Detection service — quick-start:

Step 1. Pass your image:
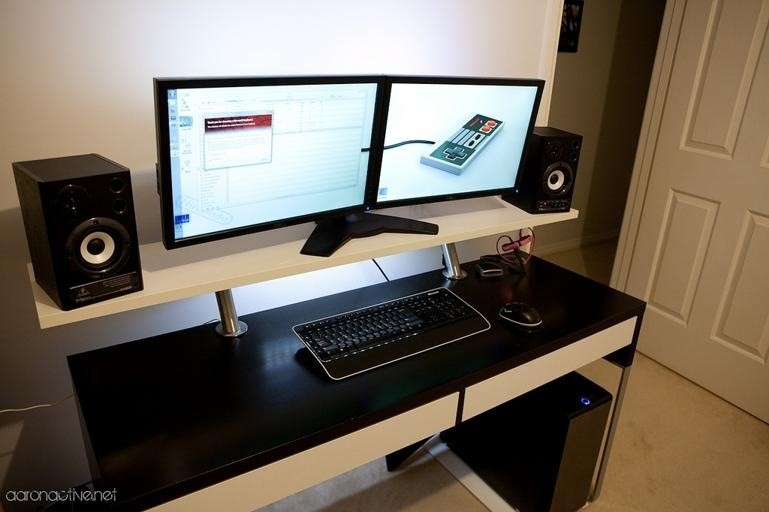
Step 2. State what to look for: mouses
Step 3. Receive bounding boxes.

[499,302,543,327]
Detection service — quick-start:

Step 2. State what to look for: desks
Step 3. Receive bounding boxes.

[26,194,647,512]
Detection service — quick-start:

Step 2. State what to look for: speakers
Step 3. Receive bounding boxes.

[12,153,143,311]
[502,127,583,214]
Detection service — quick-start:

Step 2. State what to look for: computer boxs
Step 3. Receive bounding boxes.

[440,370,613,512]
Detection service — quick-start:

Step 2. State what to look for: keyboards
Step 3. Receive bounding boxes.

[292,286,492,381]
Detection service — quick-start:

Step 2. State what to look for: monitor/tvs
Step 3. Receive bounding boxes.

[370,76,545,210]
[153,75,385,250]
